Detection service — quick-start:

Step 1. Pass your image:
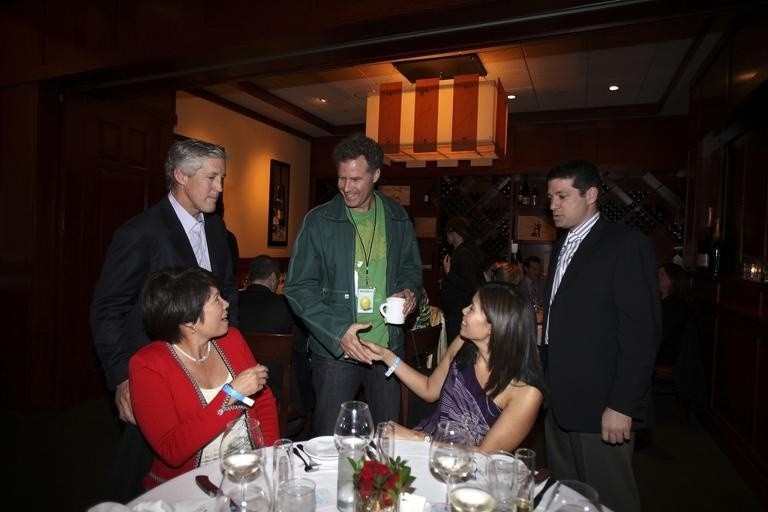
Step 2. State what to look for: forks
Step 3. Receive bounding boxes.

[296,443,320,467]
[292,448,319,473]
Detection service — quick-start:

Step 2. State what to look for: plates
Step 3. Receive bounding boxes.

[302,436,340,460]
[488,453,529,483]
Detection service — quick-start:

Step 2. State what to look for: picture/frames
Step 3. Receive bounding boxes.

[268,159,290,247]
[410,216,440,239]
[377,183,417,211]
[514,210,557,244]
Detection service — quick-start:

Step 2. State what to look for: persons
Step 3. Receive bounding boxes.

[442,219,546,347]
[344,281,544,454]
[657,262,699,374]
[89,140,238,502]
[128,268,282,492]
[542,160,662,512]
[282,133,423,435]
[239,255,316,419]
[412,288,429,331]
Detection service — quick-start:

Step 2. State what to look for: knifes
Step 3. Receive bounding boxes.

[195,476,238,512]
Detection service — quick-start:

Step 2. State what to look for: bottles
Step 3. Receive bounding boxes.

[272,183,285,229]
[692,205,723,277]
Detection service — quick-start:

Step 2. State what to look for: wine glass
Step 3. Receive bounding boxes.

[446,450,499,512]
[219,417,267,512]
[334,401,374,450]
[428,420,474,512]
[544,479,603,512]
[214,466,274,512]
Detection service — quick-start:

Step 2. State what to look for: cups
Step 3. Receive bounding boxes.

[376,423,395,465]
[485,451,518,512]
[278,477,316,512]
[336,448,363,512]
[515,447,536,512]
[272,439,294,512]
[379,296,406,325]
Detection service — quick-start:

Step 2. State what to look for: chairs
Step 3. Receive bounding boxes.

[402,324,441,429]
[649,323,709,434]
[237,333,295,435]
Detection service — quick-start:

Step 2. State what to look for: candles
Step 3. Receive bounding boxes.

[707,207,713,225]
[714,217,721,240]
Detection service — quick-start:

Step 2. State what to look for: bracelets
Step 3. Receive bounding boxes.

[385,356,401,377]
[222,384,244,402]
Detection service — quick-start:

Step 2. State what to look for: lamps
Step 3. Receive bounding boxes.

[366,53,509,169]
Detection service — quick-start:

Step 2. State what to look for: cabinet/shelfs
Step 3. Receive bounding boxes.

[688,275,768,512]
[435,172,515,313]
[595,172,690,267]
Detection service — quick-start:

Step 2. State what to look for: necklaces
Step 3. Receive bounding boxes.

[173,341,211,362]
